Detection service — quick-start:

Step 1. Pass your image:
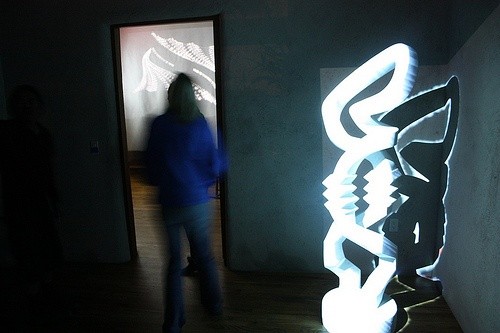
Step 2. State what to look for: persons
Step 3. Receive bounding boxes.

[128,66,229,331]
